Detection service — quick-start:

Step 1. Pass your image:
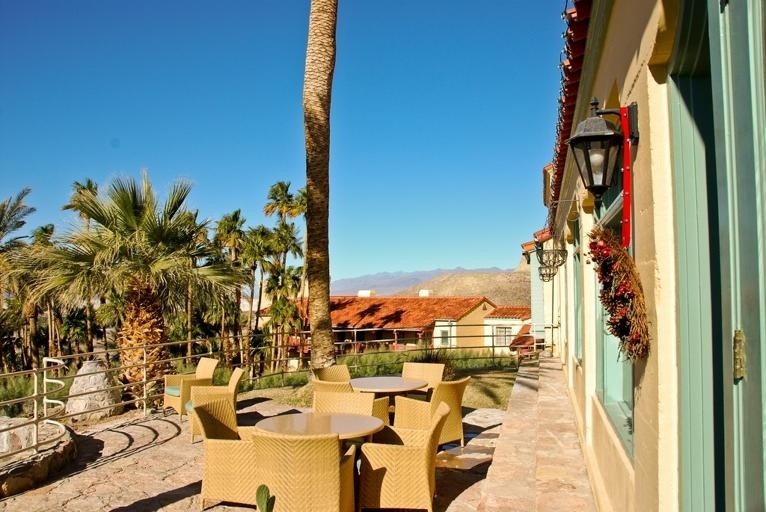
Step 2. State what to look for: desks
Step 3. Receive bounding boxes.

[254,413,383,450]
[348,377,429,407]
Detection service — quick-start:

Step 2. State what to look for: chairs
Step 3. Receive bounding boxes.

[399,361,445,399]
[312,378,391,425]
[190,399,256,512]
[185,367,245,443]
[359,402,452,512]
[249,432,356,512]
[311,364,349,381]
[393,375,471,448]
[161,356,221,423]
[310,391,374,444]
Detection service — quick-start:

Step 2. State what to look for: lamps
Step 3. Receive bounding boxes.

[567,97,639,207]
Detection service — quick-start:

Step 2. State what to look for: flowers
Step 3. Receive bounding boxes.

[584,218,650,363]
